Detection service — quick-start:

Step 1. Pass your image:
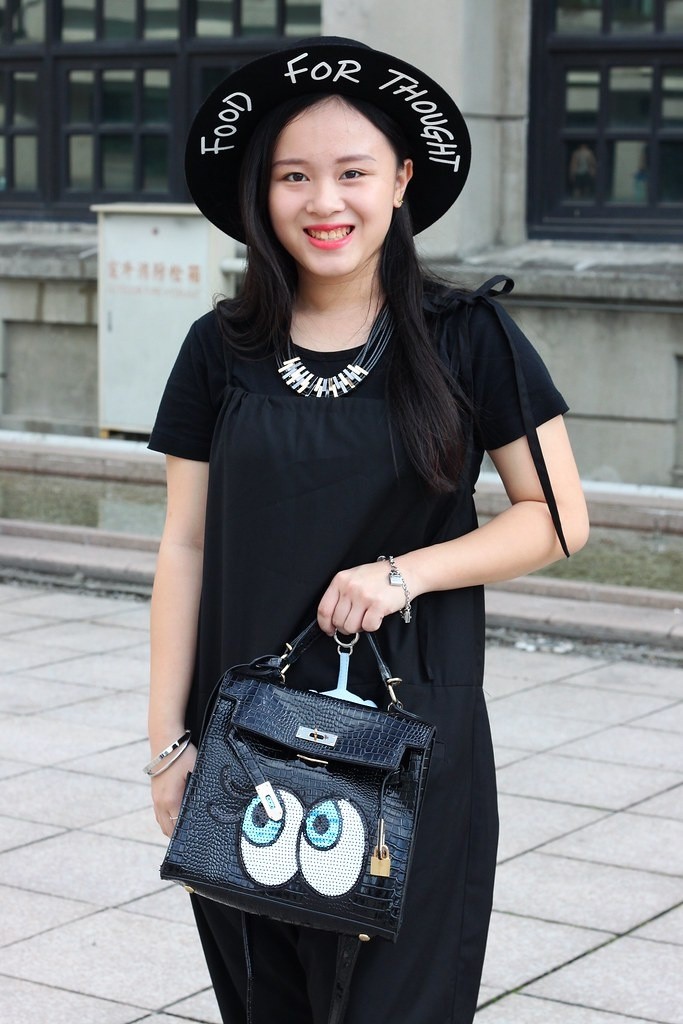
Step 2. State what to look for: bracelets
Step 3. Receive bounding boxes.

[378,553,411,624]
[143,729,192,778]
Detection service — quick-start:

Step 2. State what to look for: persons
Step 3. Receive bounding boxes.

[136,34,593,1024]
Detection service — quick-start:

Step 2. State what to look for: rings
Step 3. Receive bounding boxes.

[170,816,177,820]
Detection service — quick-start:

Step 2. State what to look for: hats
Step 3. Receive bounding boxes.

[184,36,472,247]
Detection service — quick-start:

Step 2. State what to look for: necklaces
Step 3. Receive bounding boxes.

[266,304,397,402]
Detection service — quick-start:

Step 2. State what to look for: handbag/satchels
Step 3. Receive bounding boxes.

[160,615,436,945]
